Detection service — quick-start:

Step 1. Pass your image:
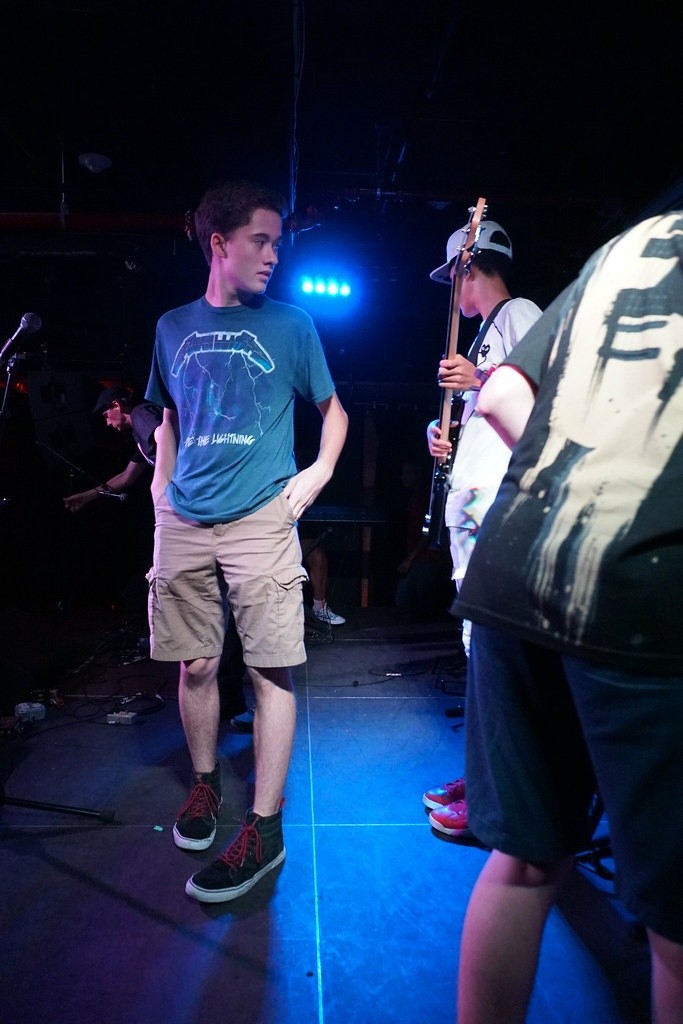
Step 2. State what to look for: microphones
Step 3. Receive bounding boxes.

[99,491,128,504]
[0,312,42,371]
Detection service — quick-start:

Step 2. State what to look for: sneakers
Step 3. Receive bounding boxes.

[172,758,223,851]
[429,799,472,836]
[422,778,465,810]
[312,602,346,625]
[184,808,286,903]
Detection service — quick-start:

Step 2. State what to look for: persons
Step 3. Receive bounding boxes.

[61,384,255,730]
[143,183,351,903]
[395,479,442,621]
[423,218,544,839]
[294,437,346,626]
[450,209,683,1024]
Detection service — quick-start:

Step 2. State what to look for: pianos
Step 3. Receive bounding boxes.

[297,502,394,528]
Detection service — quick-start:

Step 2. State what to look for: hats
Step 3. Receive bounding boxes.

[430,221,512,285]
[91,387,130,413]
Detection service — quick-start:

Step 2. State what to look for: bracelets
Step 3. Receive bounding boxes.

[94,488,100,496]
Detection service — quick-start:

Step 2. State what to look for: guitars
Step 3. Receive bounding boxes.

[419,197,489,553]
